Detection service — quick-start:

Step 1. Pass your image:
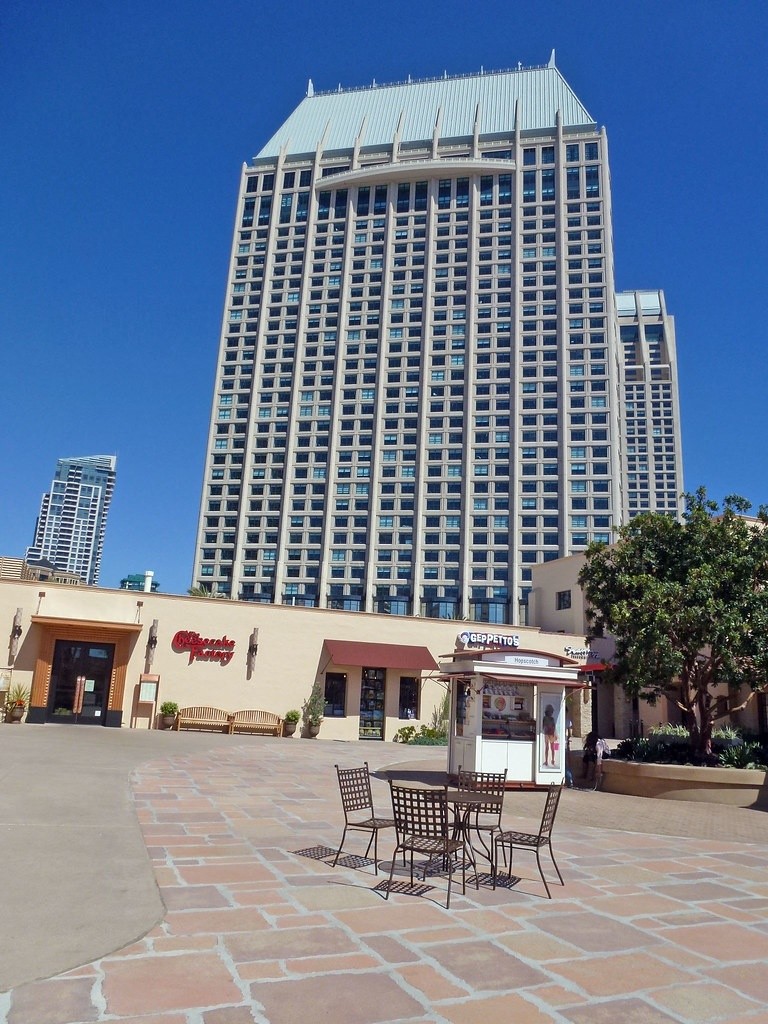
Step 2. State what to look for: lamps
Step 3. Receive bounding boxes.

[137,601,144,622]
[36,592,45,614]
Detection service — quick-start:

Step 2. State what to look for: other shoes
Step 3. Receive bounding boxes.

[543,762,548,766]
[551,761,554,765]
[576,785,593,791]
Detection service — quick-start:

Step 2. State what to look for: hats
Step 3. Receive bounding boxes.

[545,704,554,711]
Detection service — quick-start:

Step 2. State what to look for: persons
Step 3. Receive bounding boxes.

[583,732,611,790]
[565,705,574,788]
[543,704,558,766]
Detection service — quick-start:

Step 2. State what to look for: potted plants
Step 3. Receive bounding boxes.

[302,685,328,736]
[160,701,178,730]
[5,683,30,721]
[284,710,300,735]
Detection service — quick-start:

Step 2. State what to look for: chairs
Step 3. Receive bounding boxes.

[445,764,508,869]
[491,783,566,899]
[385,778,466,909]
[332,762,407,876]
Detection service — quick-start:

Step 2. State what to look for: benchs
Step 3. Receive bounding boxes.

[230,709,283,737]
[175,705,232,735]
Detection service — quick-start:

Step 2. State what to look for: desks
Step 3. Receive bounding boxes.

[423,791,503,890]
